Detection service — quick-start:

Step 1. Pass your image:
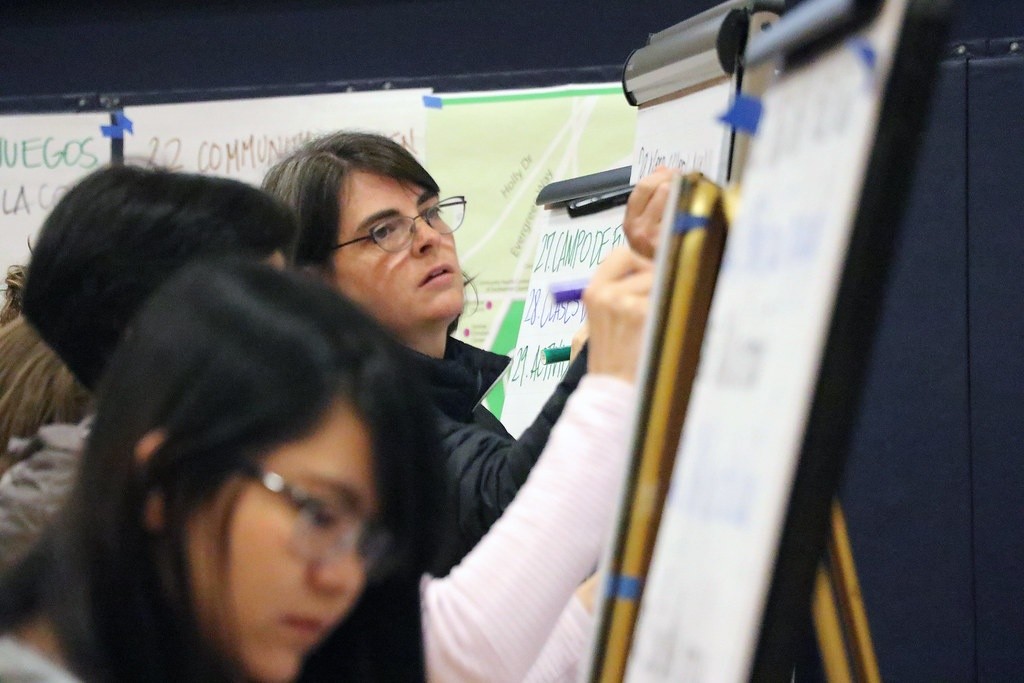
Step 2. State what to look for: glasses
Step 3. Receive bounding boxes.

[326,196,467,255]
[234,456,393,574]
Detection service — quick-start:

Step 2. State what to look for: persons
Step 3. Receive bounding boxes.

[260,132,680,576]
[0,264,437,683]
[19,162,657,683]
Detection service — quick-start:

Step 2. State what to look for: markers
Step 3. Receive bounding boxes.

[541,346,571,365]
[565,185,634,218]
[553,289,583,303]
